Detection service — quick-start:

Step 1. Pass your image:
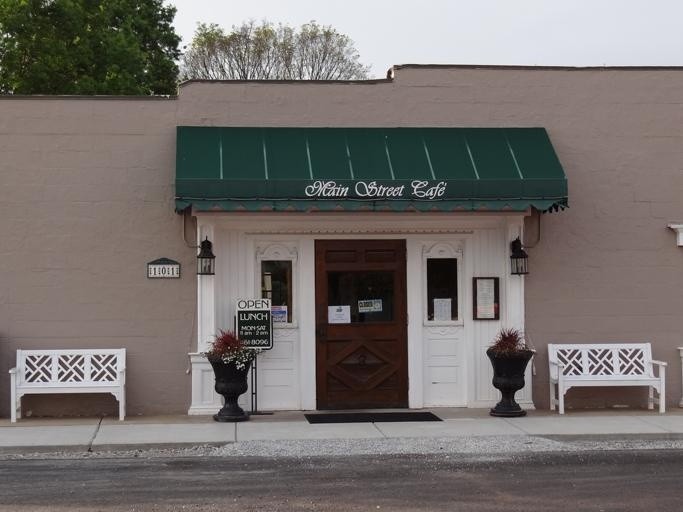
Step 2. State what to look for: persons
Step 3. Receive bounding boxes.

[337,307,342,312]
[359,302,370,307]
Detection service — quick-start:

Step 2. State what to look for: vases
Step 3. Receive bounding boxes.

[204,351,252,422]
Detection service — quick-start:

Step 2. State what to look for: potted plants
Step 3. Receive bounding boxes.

[485,326,534,416]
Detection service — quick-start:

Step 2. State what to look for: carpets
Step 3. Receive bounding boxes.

[302,411,442,423]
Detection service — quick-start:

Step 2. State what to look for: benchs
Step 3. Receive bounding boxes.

[547,342,668,413]
[7,348,131,426]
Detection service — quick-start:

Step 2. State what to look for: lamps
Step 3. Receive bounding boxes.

[196,234,216,276]
[510,236,529,275]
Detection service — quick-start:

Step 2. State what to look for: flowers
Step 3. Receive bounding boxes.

[200,328,257,372]
